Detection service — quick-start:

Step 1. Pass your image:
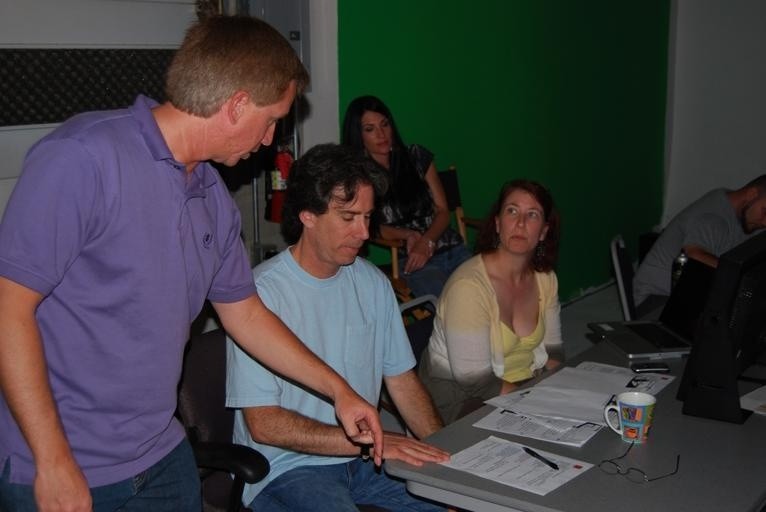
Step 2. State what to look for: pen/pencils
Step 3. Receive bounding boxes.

[522,446,559,470]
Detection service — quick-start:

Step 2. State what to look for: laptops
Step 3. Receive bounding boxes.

[588,258,716,360]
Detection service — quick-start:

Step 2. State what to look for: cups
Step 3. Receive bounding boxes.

[604,393,657,445]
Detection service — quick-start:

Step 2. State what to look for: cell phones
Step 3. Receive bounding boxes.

[631,362,669,373]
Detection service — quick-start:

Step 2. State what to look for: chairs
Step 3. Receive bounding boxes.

[400,293,439,372]
[365,164,470,319]
[610,233,636,322]
[175,324,270,512]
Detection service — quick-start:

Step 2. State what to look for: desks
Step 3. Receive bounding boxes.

[381,328,766,511]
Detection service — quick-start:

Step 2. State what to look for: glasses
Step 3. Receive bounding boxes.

[598,440,680,483]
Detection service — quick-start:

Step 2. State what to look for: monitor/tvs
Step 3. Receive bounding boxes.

[675,228,766,424]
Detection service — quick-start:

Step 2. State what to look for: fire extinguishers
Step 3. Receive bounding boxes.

[269,147,294,223]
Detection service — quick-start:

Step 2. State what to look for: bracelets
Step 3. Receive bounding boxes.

[360,439,370,462]
[420,235,439,250]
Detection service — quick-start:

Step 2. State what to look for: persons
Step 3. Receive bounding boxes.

[222,140,462,511]
[631,174,766,326]
[404,178,565,444]
[342,94,474,317]
[0,13,384,511]
